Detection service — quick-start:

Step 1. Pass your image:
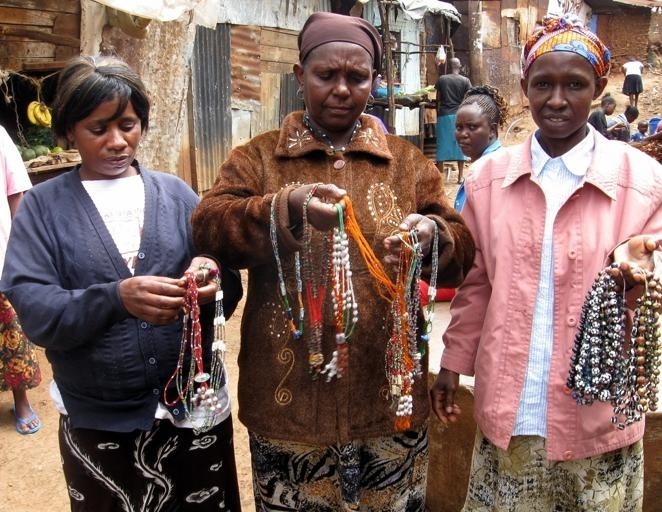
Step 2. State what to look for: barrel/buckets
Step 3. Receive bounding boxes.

[11,402,41,435]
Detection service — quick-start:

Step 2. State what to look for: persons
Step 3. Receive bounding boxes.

[429,15,661,512]
[191,9,475,511]
[0,50,244,511]
[450,85,508,211]
[587,93,626,141]
[605,105,638,143]
[619,57,645,108]
[433,55,470,183]
[0,122,45,434]
[630,121,647,144]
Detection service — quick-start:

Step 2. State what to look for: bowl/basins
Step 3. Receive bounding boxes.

[565,258,662,431]
[296,110,367,156]
[270,181,359,383]
[340,197,439,433]
[163,263,229,437]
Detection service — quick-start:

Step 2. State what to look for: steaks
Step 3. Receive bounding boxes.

[15,144,64,161]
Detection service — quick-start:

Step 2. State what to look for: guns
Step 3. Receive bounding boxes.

[27,101,52,128]
